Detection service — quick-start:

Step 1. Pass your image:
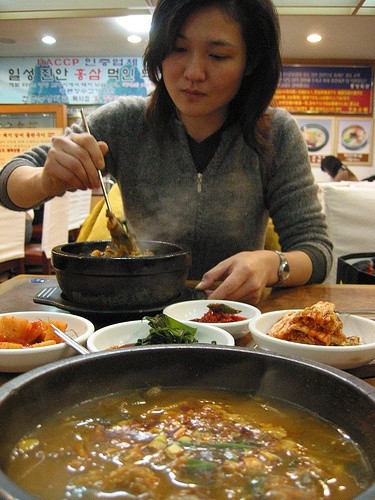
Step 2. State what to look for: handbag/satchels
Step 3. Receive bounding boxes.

[336,251,375,285]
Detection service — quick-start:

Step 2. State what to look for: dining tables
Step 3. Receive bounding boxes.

[0,274,375,386]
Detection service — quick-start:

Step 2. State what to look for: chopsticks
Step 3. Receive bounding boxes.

[50,323,90,355]
[77,106,115,218]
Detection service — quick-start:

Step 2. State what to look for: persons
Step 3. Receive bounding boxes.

[320,155,358,182]
[0,0,333,309]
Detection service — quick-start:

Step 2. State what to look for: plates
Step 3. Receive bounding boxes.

[0,311,96,374]
[87,318,235,360]
[1,343,375,500]
[161,299,261,340]
[247,310,375,370]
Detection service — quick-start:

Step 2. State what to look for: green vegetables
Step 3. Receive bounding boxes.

[178,438,370,500]
[131,313,220,346]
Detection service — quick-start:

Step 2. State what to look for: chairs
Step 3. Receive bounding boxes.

[0,180,375,285]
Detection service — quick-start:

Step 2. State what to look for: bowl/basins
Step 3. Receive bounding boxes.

[51,236,192,309]
[300,123,329,151]
[341,125,368,150]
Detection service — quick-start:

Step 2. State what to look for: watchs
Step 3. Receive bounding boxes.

[274,250,290,287]
